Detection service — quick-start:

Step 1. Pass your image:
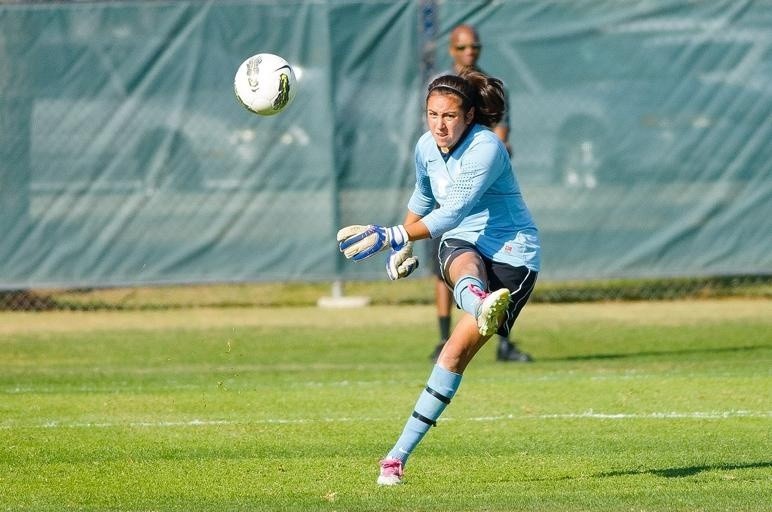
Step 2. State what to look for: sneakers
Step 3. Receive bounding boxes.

[377,455,406,487]
[475,287,512,338]
[496,340,533,363]
[428,338,448,361]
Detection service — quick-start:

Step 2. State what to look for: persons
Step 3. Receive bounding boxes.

[334,65,547,489]
[419,21,538,368]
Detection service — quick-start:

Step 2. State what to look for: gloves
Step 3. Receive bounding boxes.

[385,241,421,282]
[336,224,409,264]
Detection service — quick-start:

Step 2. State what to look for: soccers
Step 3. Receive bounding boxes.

[234,53,297,118]
